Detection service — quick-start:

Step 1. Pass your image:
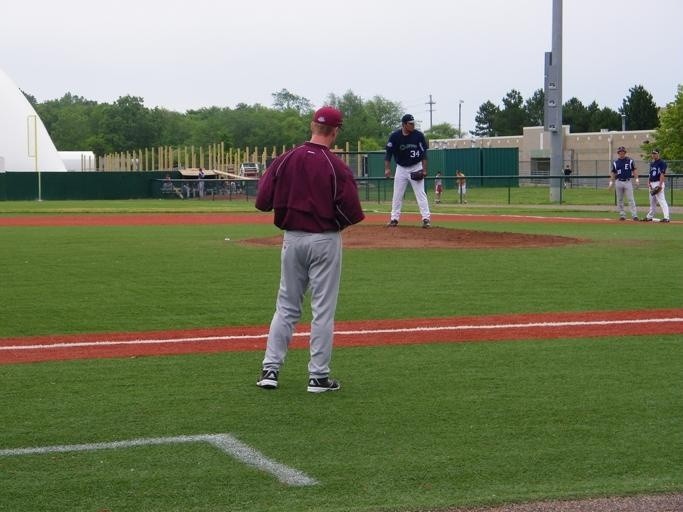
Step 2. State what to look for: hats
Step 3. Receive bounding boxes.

[402,115,416,124]
[314,107,344,131]
[652,149,659,153]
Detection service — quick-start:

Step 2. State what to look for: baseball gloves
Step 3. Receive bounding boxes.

[649,186,662,196]
[410,169,424,181]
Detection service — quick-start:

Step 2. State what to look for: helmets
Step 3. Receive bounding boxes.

[617,146,627,154]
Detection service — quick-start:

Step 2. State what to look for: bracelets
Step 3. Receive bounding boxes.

[635,178,639,183]
[609,180,613,187]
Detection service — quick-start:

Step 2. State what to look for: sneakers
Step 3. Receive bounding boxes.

[391,220,398,226]
[307,378,340,392]
[257,370,278,388]
[423,219,431,228]
[620,217,670,222]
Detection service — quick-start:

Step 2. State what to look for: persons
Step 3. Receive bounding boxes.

[163,168,241,199]
[435,172,442,203]
[641,148,671,222]
[607,145,640,221]
[252,107,365,391]
[564,166,572,188]
[456,169,467,203]
[385,113,433,228]
